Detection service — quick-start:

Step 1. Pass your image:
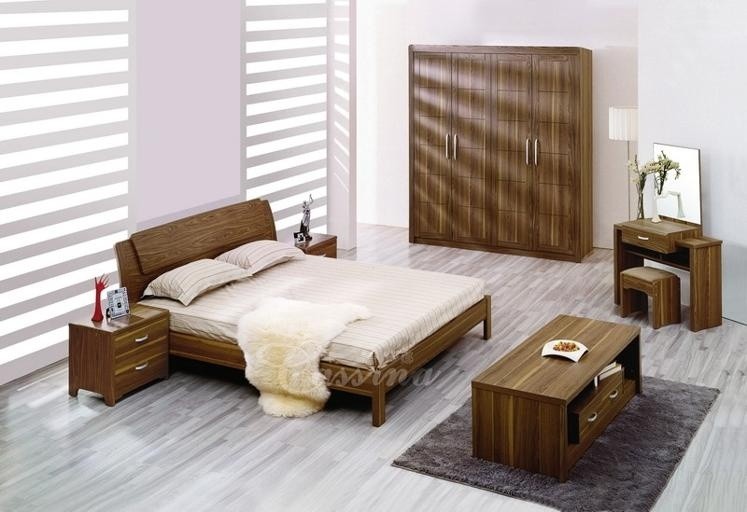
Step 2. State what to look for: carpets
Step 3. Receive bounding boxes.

[391,376,721,511]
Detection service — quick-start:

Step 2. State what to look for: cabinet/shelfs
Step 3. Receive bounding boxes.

[490,46,593,263]
[408,45,491,252]
[613,217,722,332]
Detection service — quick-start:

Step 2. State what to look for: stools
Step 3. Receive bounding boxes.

[620,266,681,328]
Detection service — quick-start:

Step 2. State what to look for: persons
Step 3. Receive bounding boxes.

[91,272,111,322]
[300,193,314,239]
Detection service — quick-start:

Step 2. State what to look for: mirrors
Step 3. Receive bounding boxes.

[653,143,702,227]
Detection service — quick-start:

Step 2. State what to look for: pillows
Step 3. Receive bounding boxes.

[141,259,252,307]
[215,240,305,274]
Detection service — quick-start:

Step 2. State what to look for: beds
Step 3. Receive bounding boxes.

[115,198,492,426]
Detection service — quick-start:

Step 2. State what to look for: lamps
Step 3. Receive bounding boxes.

[608,108,638,221]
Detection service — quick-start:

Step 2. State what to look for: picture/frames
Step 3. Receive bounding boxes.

[106,286,130,319]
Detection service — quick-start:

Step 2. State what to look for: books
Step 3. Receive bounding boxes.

[595,361,622,383]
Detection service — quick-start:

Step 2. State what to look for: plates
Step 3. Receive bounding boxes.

[541,339,589,363]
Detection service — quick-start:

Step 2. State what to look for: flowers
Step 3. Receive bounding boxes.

[654,150,682,195]
[627,153,663,219]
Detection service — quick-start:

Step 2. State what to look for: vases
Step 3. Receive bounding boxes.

[677,194,684,218]
[636,183,645,219]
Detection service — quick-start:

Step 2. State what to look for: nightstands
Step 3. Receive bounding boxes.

[280,232,337,258]
[69,302,169,406]
[471,314,643,482]
